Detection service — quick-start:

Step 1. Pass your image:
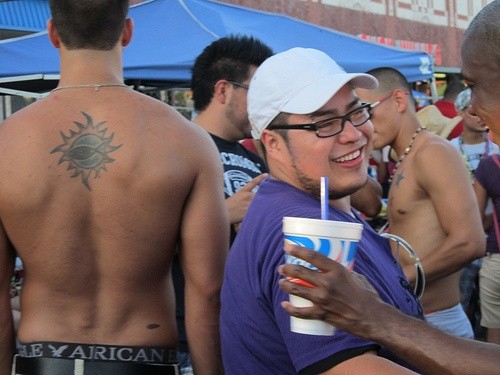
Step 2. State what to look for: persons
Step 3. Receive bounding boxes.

[170,33,500,375]
[0,0,231,375]
[278,0,500,375]
[218,47,427,375]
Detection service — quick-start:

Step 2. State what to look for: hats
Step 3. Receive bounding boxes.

[414,105,465,142]
[246,46,379,140]
[453,88,474,113]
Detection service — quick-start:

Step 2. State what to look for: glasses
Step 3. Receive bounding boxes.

[371,89,409,111]
[264,102,373,138]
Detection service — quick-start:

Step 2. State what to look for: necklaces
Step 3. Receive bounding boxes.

[48,83,128,93]
[389,127,426,183]
[454,134,490,181]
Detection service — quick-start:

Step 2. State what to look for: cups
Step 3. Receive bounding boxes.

[281,215,365,337]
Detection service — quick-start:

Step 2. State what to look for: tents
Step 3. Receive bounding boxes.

[0,0,461,105]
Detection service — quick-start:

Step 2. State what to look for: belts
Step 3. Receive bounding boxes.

[14,354,183,374]
